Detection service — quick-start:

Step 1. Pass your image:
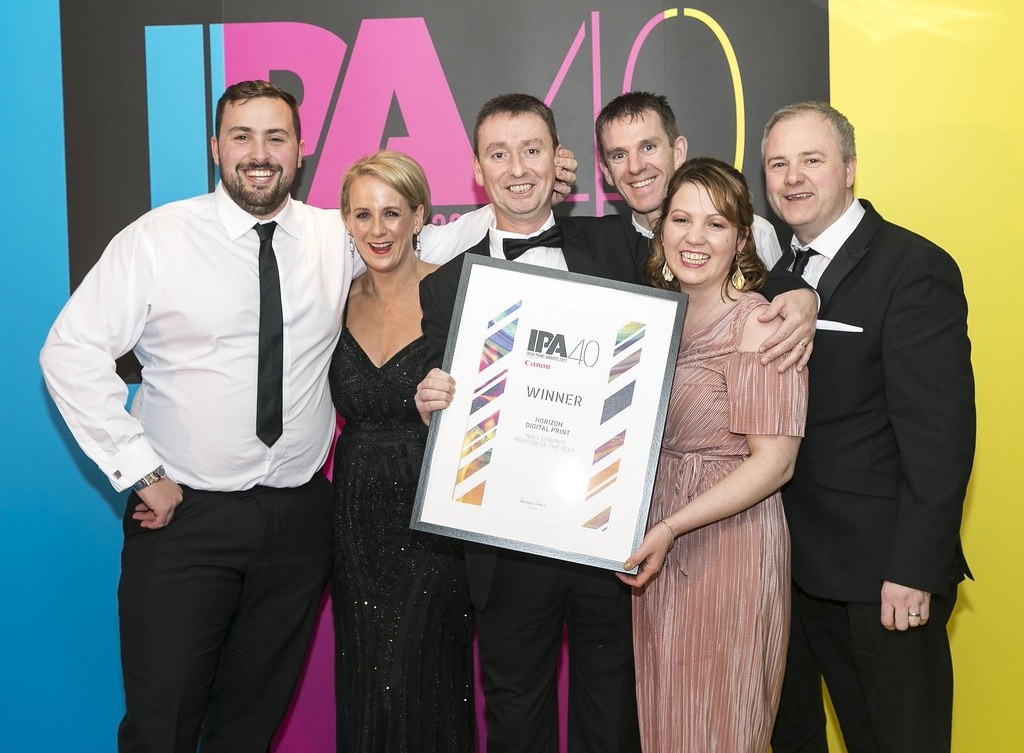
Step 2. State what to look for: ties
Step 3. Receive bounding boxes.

[252,220,284,448]
[792,247,820,276]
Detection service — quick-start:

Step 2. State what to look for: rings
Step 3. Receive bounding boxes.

[797,341,807,348]
[908,612,920,616]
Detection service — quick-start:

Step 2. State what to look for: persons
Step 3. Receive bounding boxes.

[615,158,809,753]
[595,90,829,753]
[760,102,978,753]
[39,80,579,753]
[414,93,822,753]
[327,150,480,753]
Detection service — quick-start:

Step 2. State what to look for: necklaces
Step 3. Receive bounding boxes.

[683,285,734,339]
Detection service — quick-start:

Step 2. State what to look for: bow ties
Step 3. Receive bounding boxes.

[502,224,562,261]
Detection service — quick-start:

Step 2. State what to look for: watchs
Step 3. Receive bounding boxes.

[133,465,166,491]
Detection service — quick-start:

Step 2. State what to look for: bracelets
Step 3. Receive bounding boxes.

[658,520,675,552]
